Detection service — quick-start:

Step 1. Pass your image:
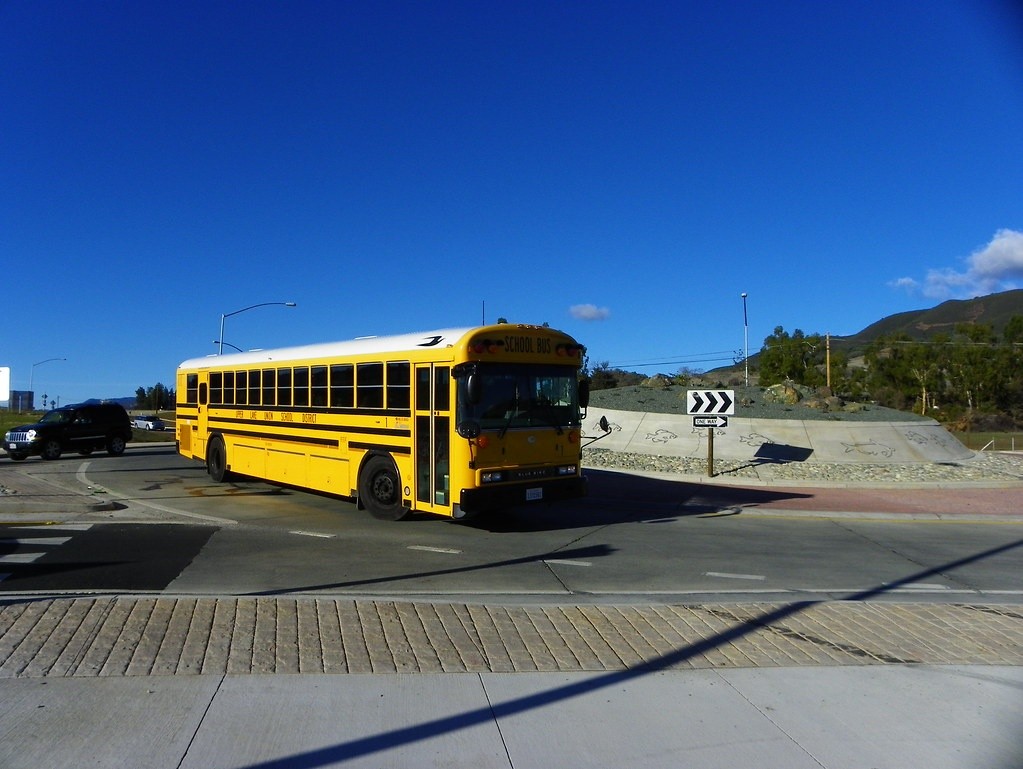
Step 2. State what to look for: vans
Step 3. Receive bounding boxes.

[134,415,166,430]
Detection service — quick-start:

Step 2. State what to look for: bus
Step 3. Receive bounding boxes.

[173,315,611,523]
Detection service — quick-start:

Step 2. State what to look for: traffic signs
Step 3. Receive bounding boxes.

[691,415,729,428]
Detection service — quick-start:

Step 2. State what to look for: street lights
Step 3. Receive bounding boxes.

[30,356,67,409]
[210,337,245,354]
[219,300,297,353]
[740,292,752,386]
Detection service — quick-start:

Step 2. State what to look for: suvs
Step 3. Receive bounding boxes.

[3,401,133,462]
